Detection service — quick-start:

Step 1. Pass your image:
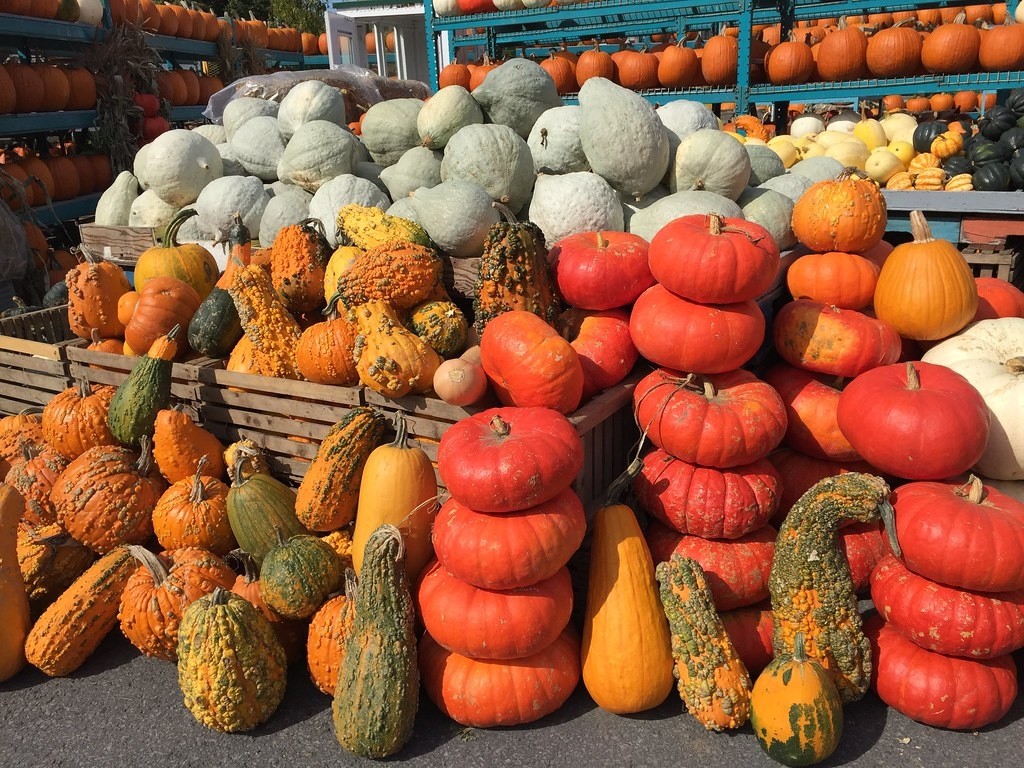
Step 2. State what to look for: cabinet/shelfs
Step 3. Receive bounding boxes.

[0,0,305,228]
[422,0,1024,247]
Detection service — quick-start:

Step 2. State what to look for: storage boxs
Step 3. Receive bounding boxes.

[961,248,1024,292]
[1,304,646,566]
[80,224,808,373]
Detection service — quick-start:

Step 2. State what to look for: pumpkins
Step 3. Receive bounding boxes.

[0,0,1024,768]
